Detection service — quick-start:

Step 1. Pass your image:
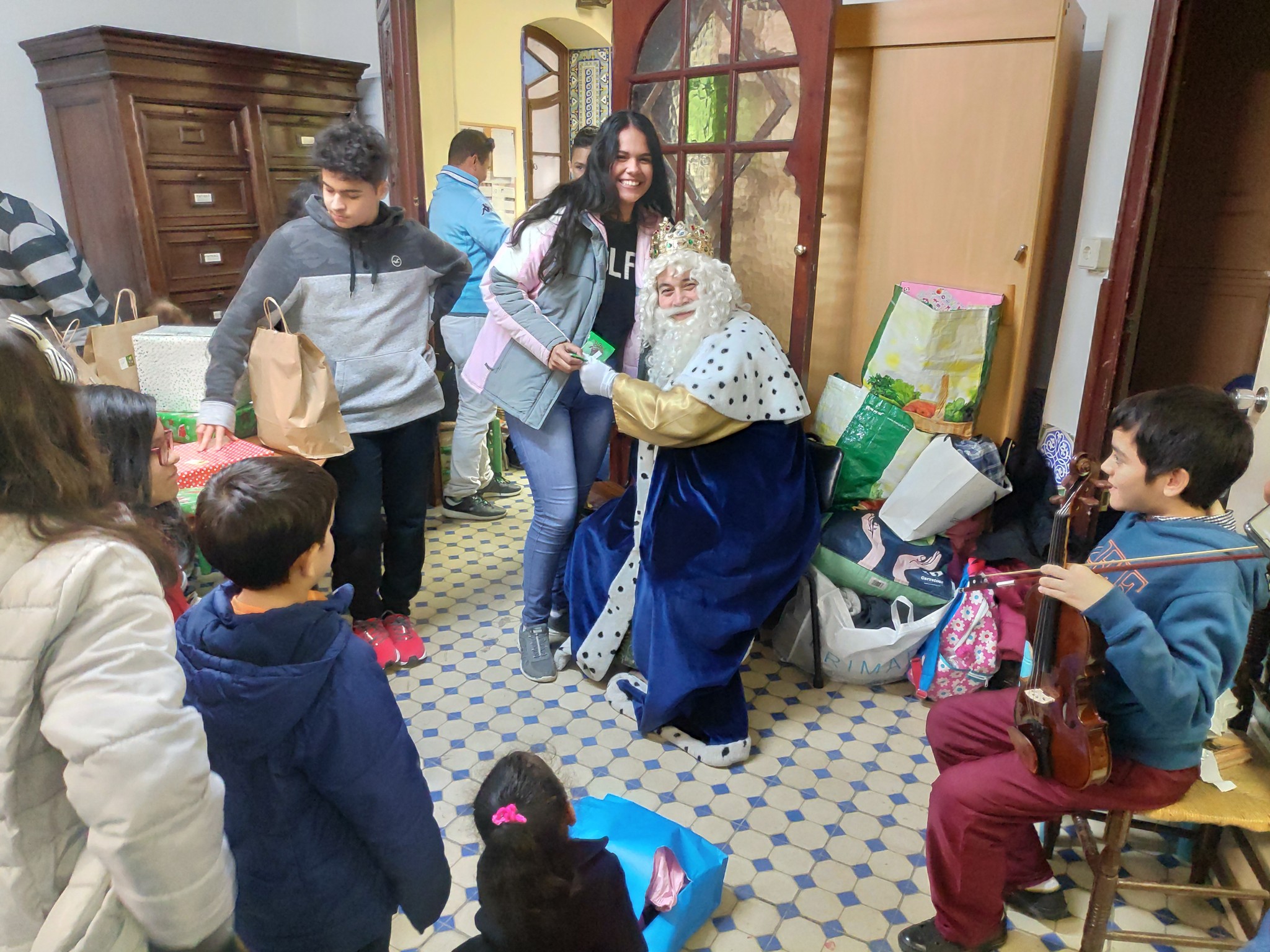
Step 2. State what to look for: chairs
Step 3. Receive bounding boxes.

[1042,563,1270,952]
[803,431,845,688]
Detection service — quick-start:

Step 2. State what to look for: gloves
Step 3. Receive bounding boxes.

[580,354,617,399]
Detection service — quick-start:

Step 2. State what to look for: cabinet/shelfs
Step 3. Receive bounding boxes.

[17,24,374,325]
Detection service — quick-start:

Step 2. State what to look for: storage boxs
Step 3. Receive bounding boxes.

[170,433,274,490]
[128,324,253,412]
[153,404,256,443]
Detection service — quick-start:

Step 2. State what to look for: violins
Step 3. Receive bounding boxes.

[1010,449,1115,793]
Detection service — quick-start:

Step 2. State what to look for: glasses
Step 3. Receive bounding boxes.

[149,429,175,465]
[485,137,495,150]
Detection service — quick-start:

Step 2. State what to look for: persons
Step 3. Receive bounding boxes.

[567,126,597,180]
[0,315,448,952]
[463,112,674,682]
[553,252,822,766]
[428,130,509,521]
[0,190,122,362]
[196,122,476,670]
[473,752,649,952]
[899,382,1270,952]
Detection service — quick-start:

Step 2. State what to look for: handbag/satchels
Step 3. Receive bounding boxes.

[861,284,1004,440]
[879,433,1014,541]
[44,317,103,387]
[82,289,160,390]
[247,296,357,460]
[810,373,935,510]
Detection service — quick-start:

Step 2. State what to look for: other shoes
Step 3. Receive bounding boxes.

[1006,885,1068,920]
[898,915,1007,951]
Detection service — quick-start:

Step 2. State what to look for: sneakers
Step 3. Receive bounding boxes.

[547,612,571,636]
[443,493,507,521]
[477,473,522,498]
[518,624,557,682]
[352,616,401,666]
[382,610,427,667]
